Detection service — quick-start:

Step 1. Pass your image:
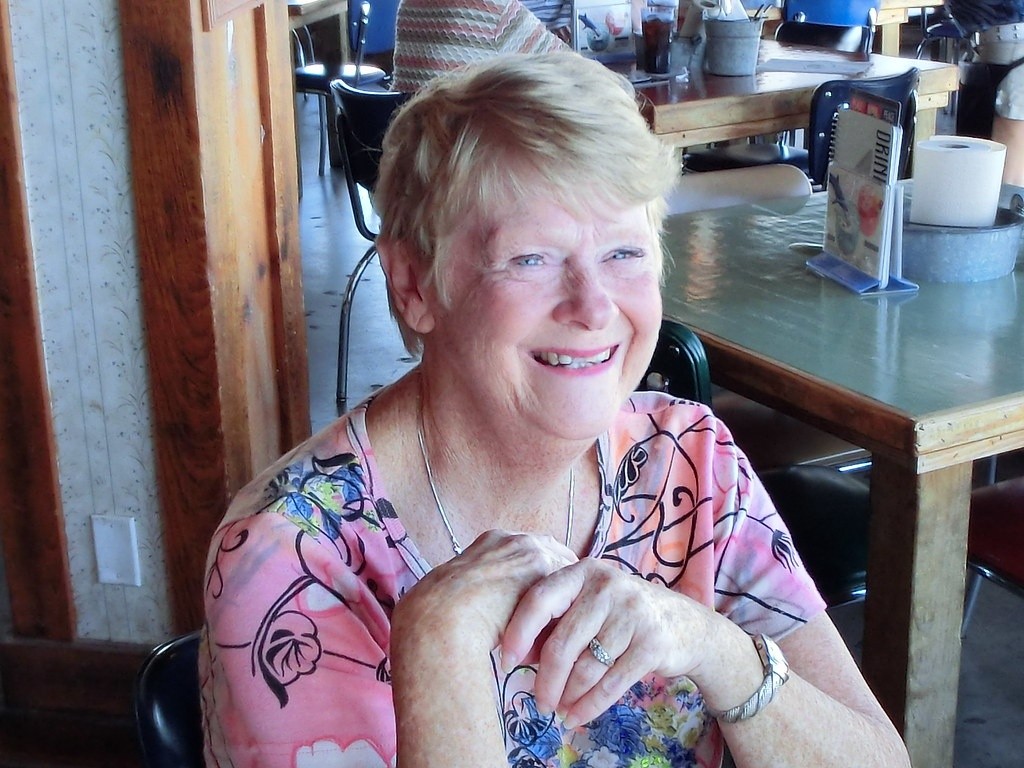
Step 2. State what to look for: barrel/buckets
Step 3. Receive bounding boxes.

[704,16,768,76]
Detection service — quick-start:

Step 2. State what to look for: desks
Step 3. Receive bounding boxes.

[571,33,963,178]
[653,174,1024,768]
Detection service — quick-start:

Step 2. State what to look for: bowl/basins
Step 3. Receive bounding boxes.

[900,206,1024,283]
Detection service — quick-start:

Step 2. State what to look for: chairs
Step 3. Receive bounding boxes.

[291,26,385,178]
[324,79,418,412]
[749,1,973,120]
[625,319,874,617]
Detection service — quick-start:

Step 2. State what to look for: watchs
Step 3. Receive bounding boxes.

[704,632,791,724]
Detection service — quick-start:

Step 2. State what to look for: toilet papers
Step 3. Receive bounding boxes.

[909,133,1007,228]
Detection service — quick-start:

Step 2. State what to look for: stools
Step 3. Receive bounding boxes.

[958,474,1020,642]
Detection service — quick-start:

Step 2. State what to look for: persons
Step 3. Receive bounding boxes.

[196,52,912,768]
[943,0,1024,188]
[387,0,573,94]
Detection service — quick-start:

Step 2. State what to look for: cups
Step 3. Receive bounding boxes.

[641,6,675,74]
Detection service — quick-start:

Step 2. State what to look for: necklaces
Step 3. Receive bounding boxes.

[416,375,574,556]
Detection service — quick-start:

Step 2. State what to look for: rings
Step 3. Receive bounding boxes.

[588,637,614,667]
